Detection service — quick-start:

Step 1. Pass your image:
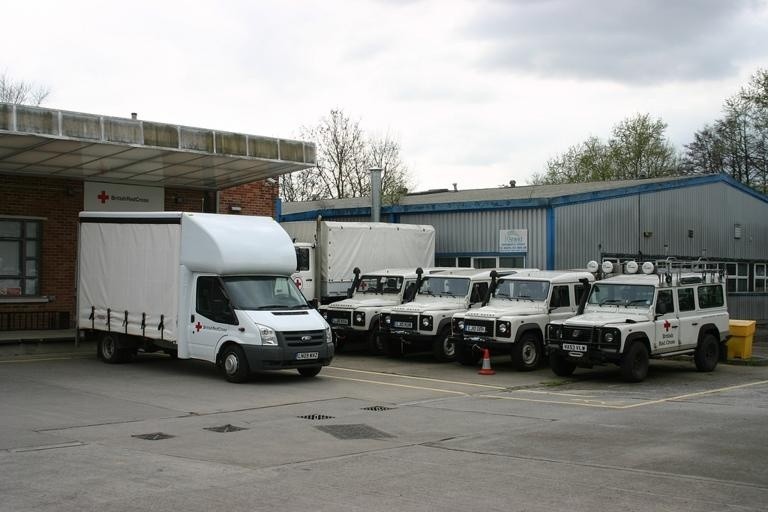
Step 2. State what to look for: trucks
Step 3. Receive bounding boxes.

[78,210,335,383]
[275,220,435,302]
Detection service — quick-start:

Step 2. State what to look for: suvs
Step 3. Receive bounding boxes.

[319,266,599,372]
[543,256,729,381]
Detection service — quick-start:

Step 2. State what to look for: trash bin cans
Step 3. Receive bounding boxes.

[727,319,756,360]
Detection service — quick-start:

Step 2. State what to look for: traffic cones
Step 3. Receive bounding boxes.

[479,349,495,374]
